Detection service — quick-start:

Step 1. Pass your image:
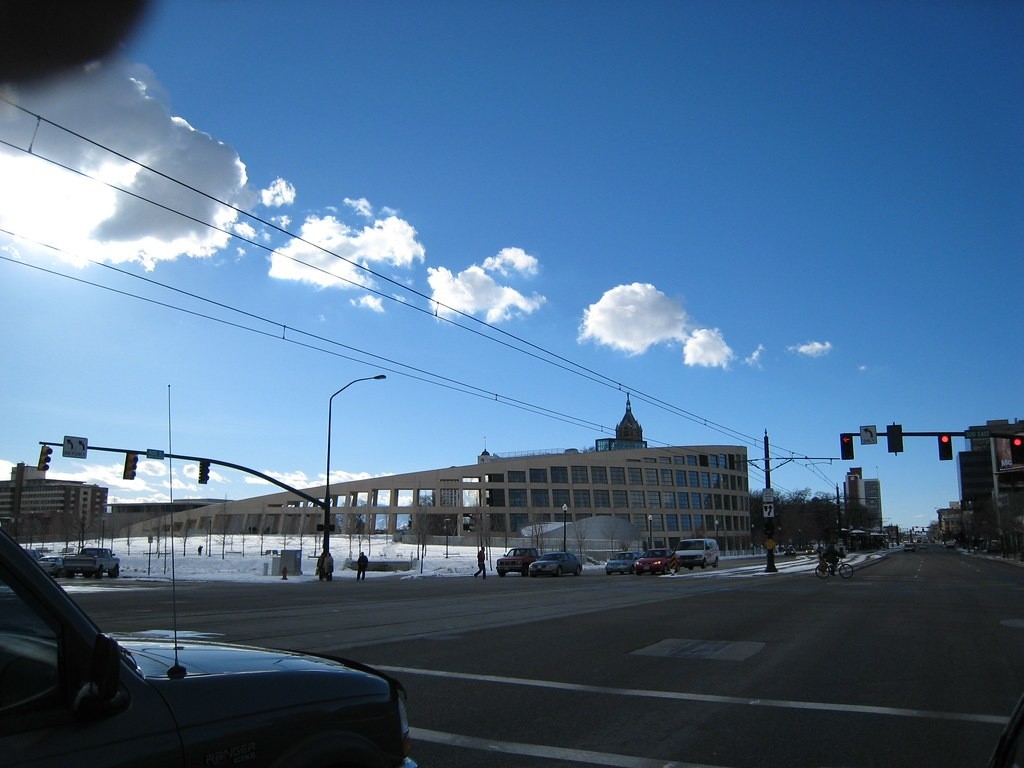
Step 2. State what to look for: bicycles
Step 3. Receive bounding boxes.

[816,555,854,578]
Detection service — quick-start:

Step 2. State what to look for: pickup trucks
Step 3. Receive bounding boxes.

[64,548,120,579]
[496,547,542,577]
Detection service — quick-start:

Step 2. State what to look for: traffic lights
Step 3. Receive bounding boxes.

[37,445,53,471]
[124,452,138,479]
[1010,437,1024,463]
[840,434,853,460]
[939,433,953,459]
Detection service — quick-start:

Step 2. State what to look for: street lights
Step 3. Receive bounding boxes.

[751,523,803,555]
[322,373,387,554]
[715,520,718,544]
[648,514,653,549]
[562,503,568,551]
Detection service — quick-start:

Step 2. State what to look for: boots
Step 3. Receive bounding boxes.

[829,569,836,577]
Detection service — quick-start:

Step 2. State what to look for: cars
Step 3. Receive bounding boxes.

[0,525,418,768]
[674,538,719,568]
[528,552,582,577]
[605,552,641,575]
[946,541,955,549]
[36,556,66,578]
[635,547,680,575]
[904,543,915,552]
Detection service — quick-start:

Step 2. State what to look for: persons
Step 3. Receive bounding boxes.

[357,552,368,581]
[822,542,846,577]
[316,551,334,581]
[473,546,486,580]
